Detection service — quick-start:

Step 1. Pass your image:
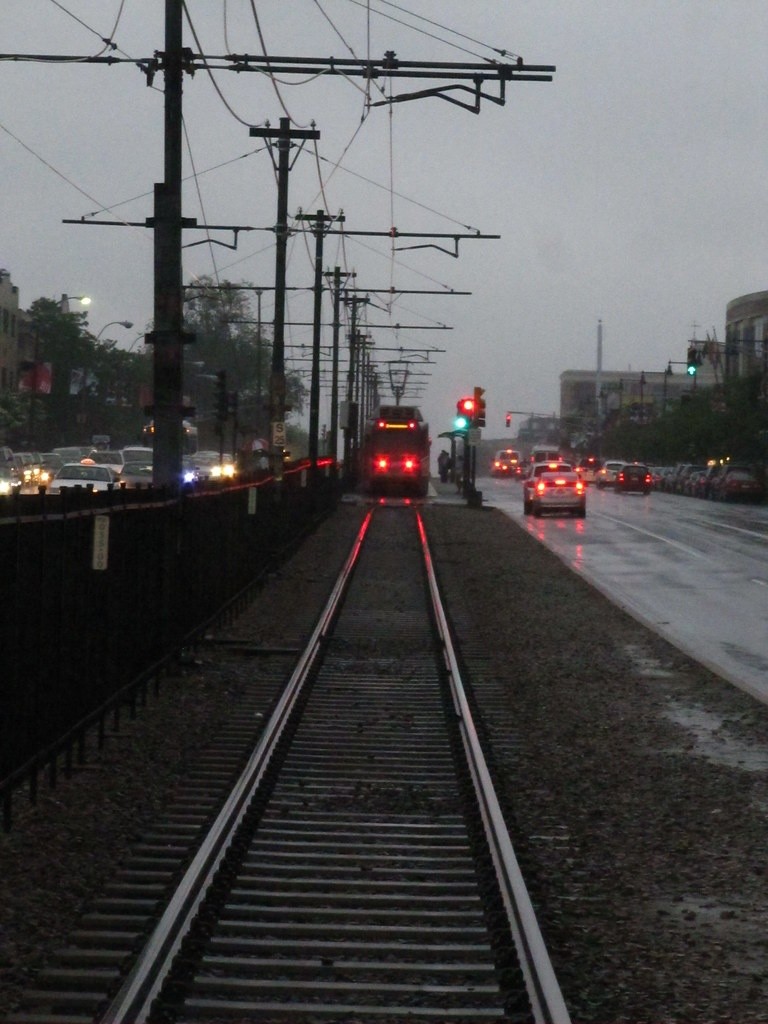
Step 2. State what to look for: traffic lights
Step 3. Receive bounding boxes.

[475,386,486,429]
[506,414,510,427]
[460,399,475,416]
[686,347,697,376]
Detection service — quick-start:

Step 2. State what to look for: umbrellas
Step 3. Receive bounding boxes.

[244,437,268,452]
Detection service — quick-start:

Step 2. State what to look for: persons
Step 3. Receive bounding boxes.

[438,450,451,482]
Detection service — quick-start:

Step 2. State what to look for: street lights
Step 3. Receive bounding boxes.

[80,320,133,446]
[26,296,92,451]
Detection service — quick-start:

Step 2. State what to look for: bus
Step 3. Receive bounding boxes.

[366,359,433,497]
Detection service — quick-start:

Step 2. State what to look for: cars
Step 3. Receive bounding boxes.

[596,459,628,490]
[648,462,763,504]
[489,443,573,514]
[0,441,270,497]
[613,462,653,496]
[575,455,603,486]
[528,471,589,519]
